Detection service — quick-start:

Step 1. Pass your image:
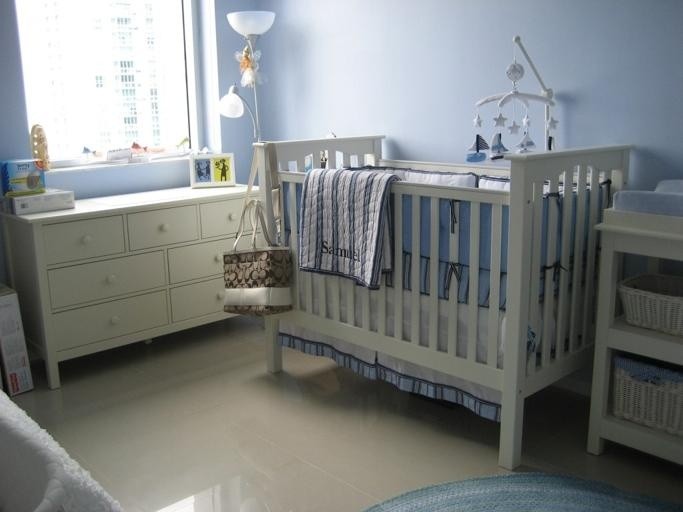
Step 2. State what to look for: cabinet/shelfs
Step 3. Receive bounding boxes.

[587,204,683,467]
[2,183,274,390]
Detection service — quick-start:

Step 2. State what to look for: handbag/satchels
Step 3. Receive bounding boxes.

[222,200,293,315]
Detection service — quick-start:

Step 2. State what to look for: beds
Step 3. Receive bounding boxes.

[253,133,632,471]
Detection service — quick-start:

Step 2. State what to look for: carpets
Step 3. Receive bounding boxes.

[364,471,683,510]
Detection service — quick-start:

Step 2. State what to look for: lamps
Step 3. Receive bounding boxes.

[215,9,276,143]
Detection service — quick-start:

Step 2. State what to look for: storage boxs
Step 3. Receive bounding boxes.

[1,289,34,397]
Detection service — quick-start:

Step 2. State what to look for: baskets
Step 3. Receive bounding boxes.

[618,272,682,335]
[612,356,683,436]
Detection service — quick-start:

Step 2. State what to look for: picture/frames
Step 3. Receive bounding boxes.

[189,153,236,188]
